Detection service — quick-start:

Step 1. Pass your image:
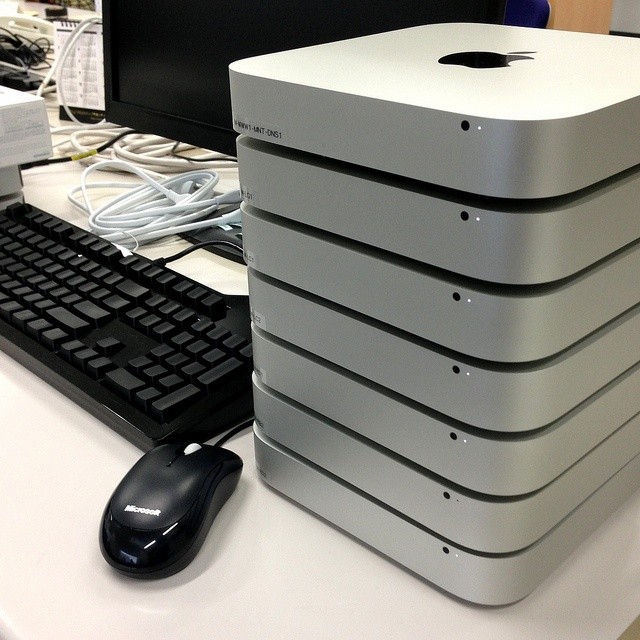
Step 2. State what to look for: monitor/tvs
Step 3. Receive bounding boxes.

[101,3,530,157]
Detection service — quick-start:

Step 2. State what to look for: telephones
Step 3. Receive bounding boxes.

[0,12,54,45]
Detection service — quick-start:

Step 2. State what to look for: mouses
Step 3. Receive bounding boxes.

[100,441,243,579]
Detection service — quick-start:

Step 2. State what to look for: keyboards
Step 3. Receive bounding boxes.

[0,200,252,440]
